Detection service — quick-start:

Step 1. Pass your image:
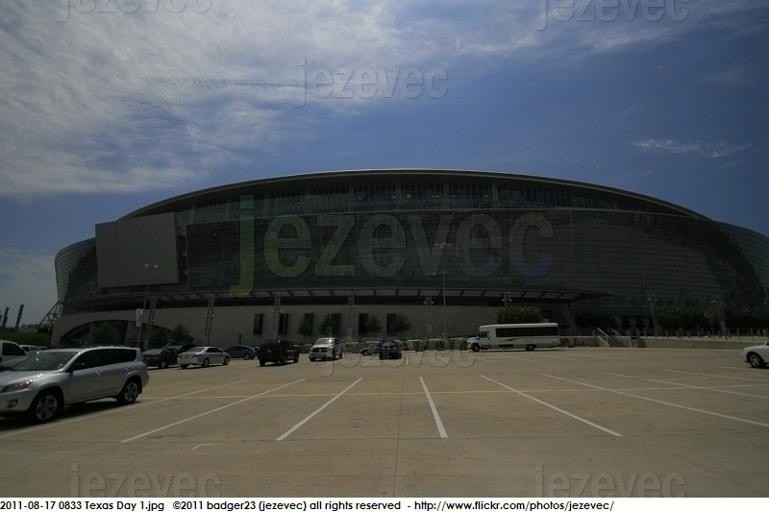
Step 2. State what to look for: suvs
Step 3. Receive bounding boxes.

[260,338,300,366]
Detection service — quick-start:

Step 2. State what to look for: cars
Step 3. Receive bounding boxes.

[0,339,148,423]
[177,344,230,368]
[310,337,343,361]
[743,339,769,368]
[143,343,198,368]
[226,345,259,360]
[360,338,402,359]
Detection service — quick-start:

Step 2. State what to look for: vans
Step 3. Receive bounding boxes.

[466,322,561,351]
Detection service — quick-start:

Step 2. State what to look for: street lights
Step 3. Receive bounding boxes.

[434,241,452,339]
[136,262,159,345]
